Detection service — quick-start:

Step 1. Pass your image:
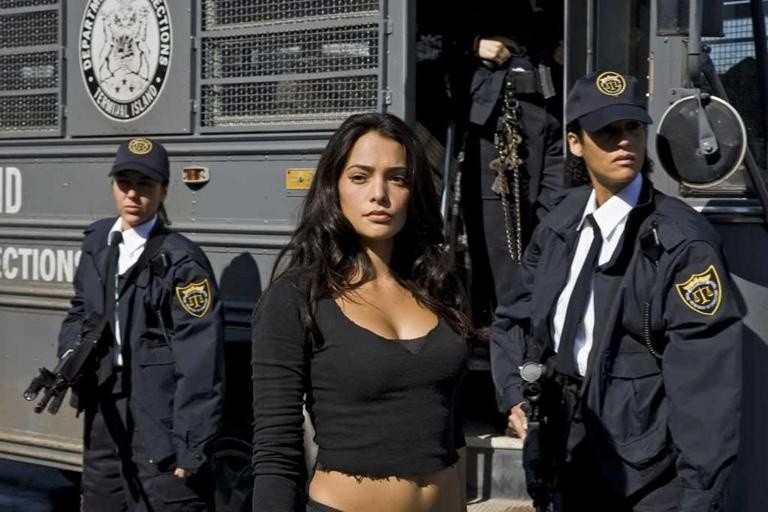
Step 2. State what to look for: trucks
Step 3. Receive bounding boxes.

[1,2,767,512]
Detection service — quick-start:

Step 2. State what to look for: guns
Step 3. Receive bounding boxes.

[479,10,564,99]
[440,2,467,221]
[25,312,104,414]
[522,329,550,502]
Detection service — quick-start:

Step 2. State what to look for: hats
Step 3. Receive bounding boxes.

[569,72,652,133]
[106,139,170,182]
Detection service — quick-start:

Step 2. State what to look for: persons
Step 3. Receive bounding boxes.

[488,70,746,511]
[250,111,554,512]
[413,1,565,430]
[55,138,226,510]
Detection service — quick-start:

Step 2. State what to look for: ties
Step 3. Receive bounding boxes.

[556,215,602,381]
[97,232,120,383]
